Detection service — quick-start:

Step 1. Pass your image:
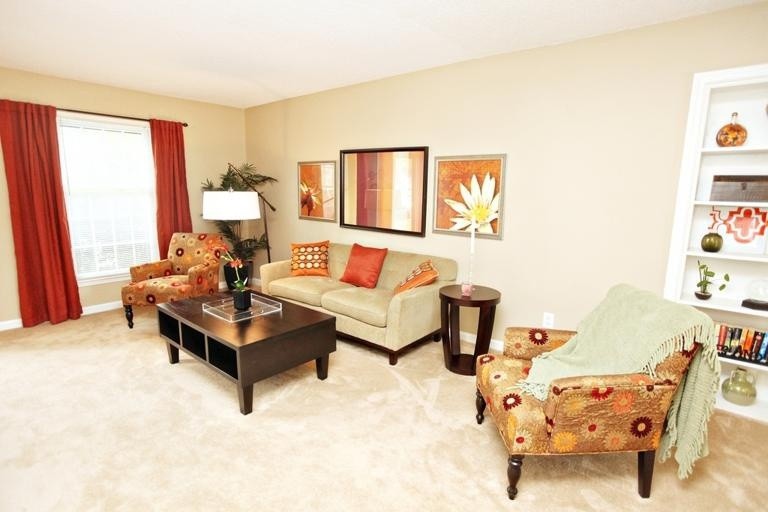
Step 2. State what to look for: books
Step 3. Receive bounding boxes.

[711,320,768,367]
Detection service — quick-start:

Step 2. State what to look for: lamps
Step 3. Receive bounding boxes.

[203,162,276,263]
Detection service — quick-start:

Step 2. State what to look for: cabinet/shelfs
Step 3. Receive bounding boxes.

[663,62,768,426]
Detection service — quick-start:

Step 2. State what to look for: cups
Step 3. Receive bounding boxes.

[461,267,473,299]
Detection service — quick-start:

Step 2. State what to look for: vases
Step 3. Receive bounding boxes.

[232,289,251,310]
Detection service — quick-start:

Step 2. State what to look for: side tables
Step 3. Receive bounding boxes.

[439,285,501,375]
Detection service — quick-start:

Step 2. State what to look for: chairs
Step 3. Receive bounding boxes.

[121,232,222,329]
[475,283,703,500]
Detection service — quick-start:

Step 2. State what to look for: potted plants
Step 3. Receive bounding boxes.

[695,259,729,299]
[200,162,279,289]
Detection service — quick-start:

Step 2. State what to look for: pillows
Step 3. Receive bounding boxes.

[338,244,388,289]
[393,259,439,295]
[290,240,330,276]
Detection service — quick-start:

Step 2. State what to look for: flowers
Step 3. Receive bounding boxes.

[220,243,249,290]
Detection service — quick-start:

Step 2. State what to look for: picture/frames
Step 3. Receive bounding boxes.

[339,146,429,237]
[297,161,337,223]
[432,154,507,240]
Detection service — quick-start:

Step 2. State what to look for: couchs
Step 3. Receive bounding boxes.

[260,243,458,365]
